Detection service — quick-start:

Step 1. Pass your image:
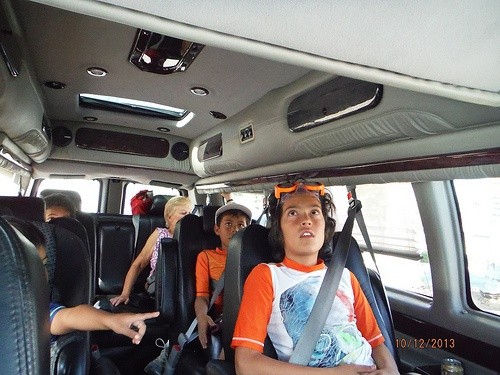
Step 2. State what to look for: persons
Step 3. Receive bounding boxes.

[109,196,195,306]
[0,215,160,345]
[231,182,401,374]
[195,204,258,361]
[43,194,77,221]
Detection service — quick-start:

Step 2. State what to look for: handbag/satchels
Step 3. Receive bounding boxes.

[130,190,153,215]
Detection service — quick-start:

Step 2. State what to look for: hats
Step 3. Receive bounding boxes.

[215,202,252,224]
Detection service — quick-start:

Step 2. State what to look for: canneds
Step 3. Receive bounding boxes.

[441,358,464,375]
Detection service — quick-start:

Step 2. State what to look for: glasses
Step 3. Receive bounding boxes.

[274,177,325,209]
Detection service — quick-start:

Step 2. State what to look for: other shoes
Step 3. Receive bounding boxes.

[143,337,171,375]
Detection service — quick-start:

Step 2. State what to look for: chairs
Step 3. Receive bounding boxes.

[0,190,401,375]
